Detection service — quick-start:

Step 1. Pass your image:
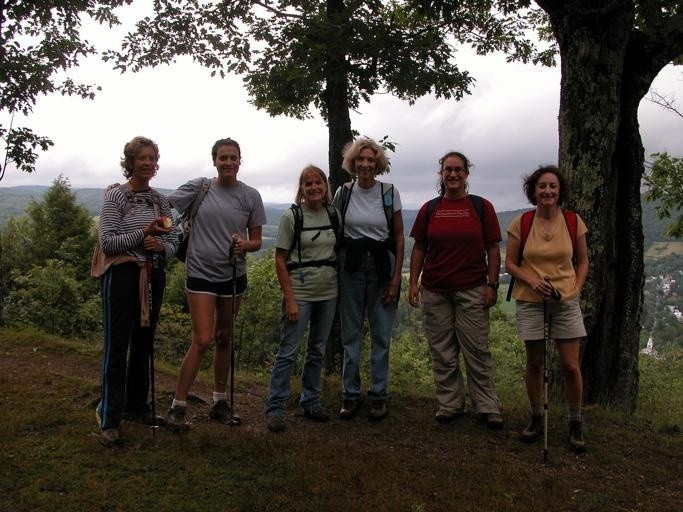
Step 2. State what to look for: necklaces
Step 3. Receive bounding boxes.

[129,179,148,191]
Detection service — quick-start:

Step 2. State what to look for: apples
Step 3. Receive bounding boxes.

[157,216,173,229]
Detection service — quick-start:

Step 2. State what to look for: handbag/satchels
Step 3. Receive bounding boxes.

[173,178,210,263]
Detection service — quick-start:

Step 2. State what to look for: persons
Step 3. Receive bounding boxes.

[89,137,181,445]
[265,166,343,432]
[408,152,503,431]
[331,140,405,422]
[505,165,590,452]
[104,138,267,426]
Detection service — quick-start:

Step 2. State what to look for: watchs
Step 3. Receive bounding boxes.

[487,281,500,289]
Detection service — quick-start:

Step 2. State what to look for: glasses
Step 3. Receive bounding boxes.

[441,167,464,173]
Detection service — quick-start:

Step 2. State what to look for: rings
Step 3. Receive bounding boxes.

[235,244,237,248]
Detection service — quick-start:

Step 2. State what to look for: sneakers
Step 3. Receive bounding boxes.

[266,415,285,432]
[485,413,503,428]
[135,411,165,426]
[339,400,358,417]
[567,421,584,452]
[164,405,195,430]
[367,400,386,421]
[101,428,121,448]
[295,405,329,422]
[521,415,544,443]
[435,409,453,421]
[208,400,240,425]
